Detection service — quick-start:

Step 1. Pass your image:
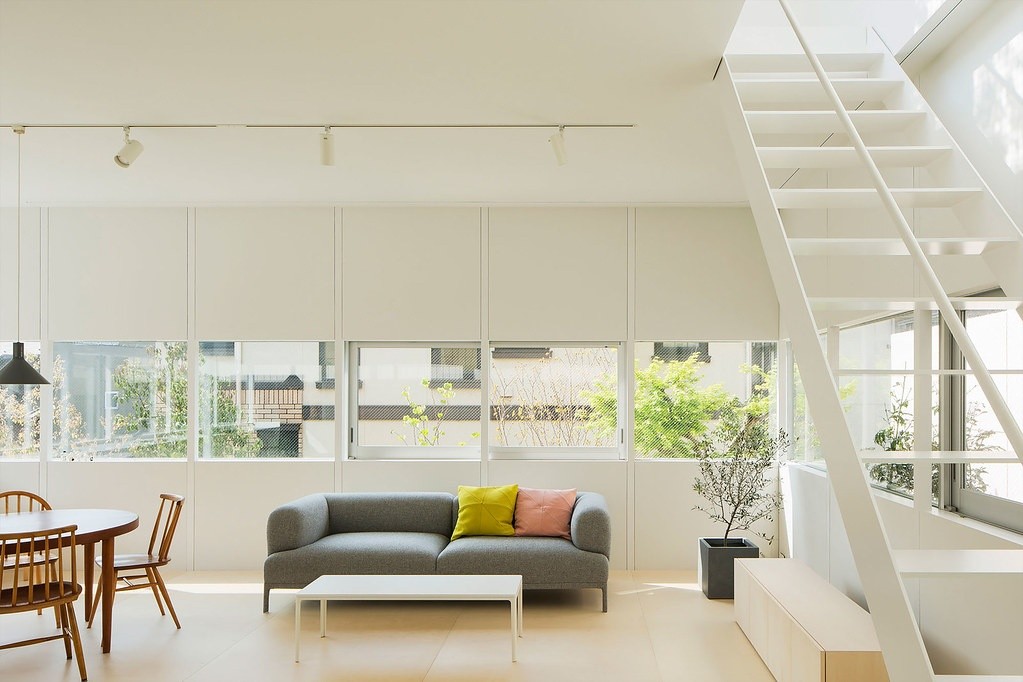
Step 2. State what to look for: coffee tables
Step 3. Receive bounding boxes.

[294,575,522,665]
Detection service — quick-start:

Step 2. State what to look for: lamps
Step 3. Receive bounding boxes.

[113,126,144,168]
[319,125,336,166]
[548,125,571,167]
[0,126,51,385]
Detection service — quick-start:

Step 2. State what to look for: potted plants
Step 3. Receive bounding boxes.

[689,411,799,600]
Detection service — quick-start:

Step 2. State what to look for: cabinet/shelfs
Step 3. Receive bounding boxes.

[733,558,889,682]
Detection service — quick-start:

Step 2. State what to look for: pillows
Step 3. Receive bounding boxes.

[451,484,518,539]
[513,486,576,540]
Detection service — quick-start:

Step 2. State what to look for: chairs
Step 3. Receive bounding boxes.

[0,525,88,682]
[0,491,61,629]
[86,493,186,647]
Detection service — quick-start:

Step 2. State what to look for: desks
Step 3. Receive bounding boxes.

[0,508,139,654]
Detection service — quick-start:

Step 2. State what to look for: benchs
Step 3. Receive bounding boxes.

[262,484,612,615]
